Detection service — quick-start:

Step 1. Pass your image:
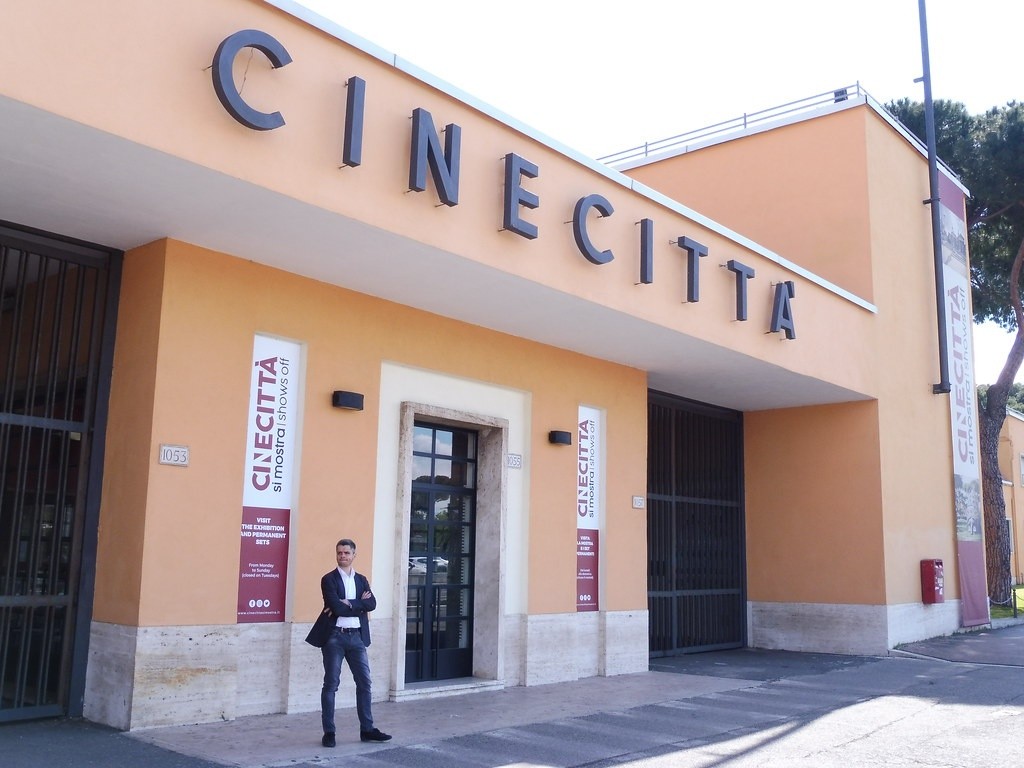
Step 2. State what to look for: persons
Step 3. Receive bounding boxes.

[303,538,393,749]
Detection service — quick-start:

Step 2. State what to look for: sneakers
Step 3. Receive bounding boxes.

[322,732,336,747]
[360,728,392,743]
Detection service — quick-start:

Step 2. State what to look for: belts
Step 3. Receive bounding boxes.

[334,626,362,633]
